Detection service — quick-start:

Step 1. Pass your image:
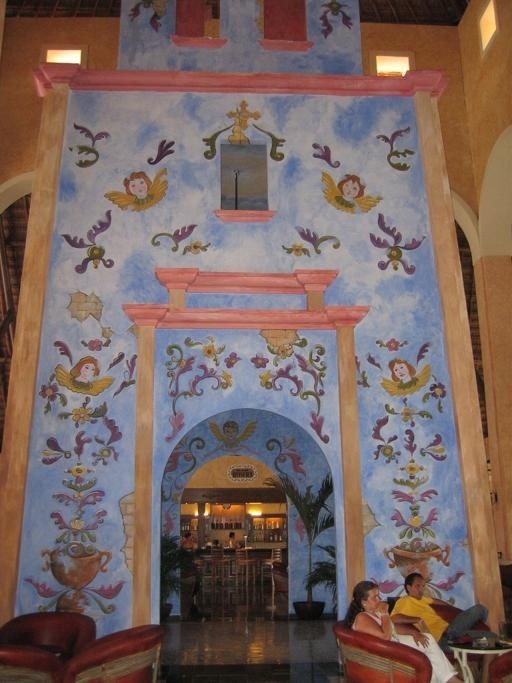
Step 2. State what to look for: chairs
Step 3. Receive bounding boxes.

[332,604,512,683]
[1,612,167,683]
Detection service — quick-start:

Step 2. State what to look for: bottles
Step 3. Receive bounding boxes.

[254,521,282,542]
[211,519,243,529]
[181,522,191,531]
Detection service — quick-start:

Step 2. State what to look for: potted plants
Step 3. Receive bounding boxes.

[161,534,202,622]
[262,471,334,620]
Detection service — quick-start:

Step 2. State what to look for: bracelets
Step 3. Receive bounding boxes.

[380,612,389,617]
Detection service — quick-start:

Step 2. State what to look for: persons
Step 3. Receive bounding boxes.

[228,532,240,548]
[342,580,466,683]
[182,532,197,568]
[389,573,497,650]
[204,539,219,572]
